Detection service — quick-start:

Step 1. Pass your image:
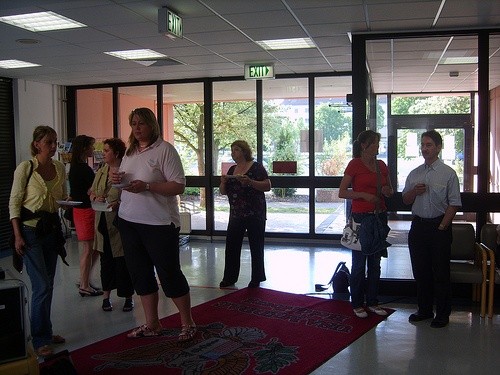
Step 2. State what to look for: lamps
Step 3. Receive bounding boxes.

[104,47,165,62]
[0,58,42,70]
[0,10,88,35]
[255,36,317,51]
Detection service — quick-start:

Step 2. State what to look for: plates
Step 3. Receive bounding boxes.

[56,201,83,205]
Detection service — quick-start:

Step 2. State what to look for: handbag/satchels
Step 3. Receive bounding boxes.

[333,263,351,293]
[64,209,72,220]
[341,219,362,251]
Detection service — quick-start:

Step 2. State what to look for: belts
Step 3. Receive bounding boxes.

[412,216,443,223]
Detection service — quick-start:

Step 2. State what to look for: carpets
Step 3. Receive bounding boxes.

[36,287,397,375]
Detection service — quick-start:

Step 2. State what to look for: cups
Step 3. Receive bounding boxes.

[110,167,127,188]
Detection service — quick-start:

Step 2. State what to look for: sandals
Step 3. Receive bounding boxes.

[178,322,197,342]
[128,324,164,337]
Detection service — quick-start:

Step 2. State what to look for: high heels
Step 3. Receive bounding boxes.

[79,283,104,297]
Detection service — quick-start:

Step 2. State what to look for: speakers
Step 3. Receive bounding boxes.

[0,281,30,365]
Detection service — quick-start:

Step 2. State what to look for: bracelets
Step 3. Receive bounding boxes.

[439,224,445,228]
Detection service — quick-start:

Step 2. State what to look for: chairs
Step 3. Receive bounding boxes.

[478,224,500,318]
[447,223,488,318]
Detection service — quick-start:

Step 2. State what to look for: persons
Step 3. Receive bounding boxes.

[68,134,104,297]
[218,140,272,288]
[339,130,395,317]
[85,137,136,312]
[8,126,75,357]
[402,130,462,328]
[110,107,198,343]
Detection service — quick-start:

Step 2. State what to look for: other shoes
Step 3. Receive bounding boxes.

[51,332,65,344]
[368,305,387,316]
[409,312,435,322]
[353,306,367,318]
[431,316,449,327]
[102,299,112,311]
[36,345,54,357]
[220,278,235,287]
[123,299,134,311]
[248,280,259,287]
